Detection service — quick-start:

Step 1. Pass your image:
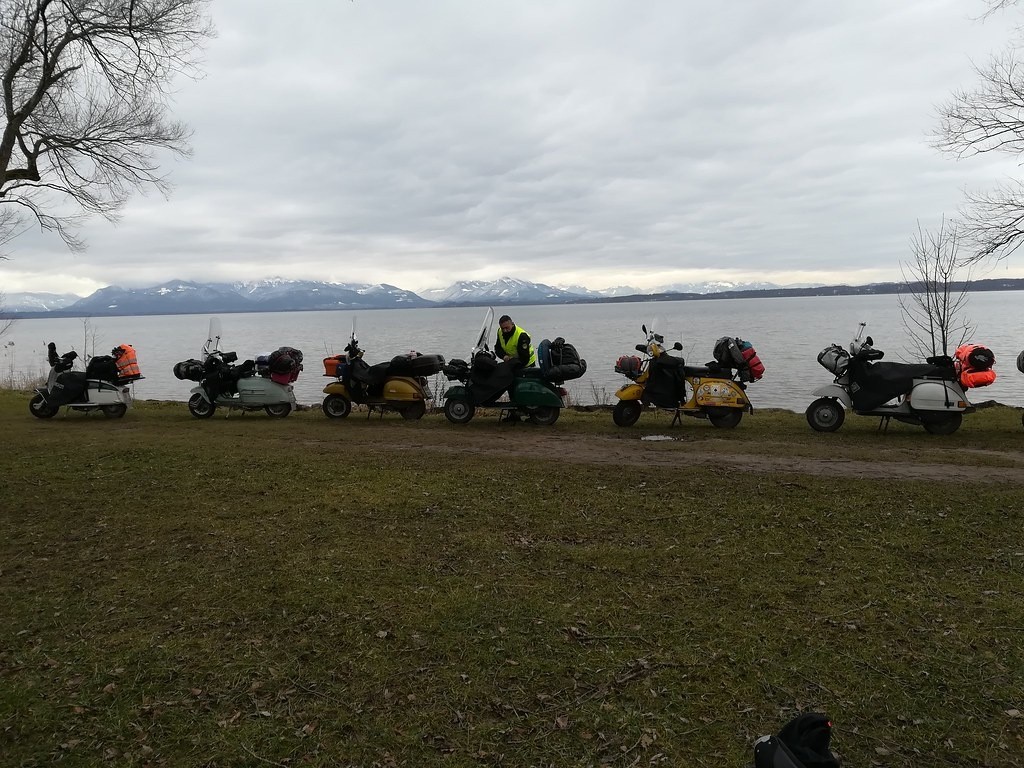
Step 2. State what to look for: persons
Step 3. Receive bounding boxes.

[493,315,536,422]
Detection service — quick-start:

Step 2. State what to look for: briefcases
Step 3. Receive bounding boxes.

[412,354,446,375]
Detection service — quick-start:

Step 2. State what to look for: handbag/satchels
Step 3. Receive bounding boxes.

[616,356,642,372]
[543,338,586,382]
[818,343,850,376]
[86,355,118,382]
[954,345,996,388]
[346,359,383,403]
[173,359,205,380]
[713,337,765,383]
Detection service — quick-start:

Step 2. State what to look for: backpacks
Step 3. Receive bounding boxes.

[648,356,686,408]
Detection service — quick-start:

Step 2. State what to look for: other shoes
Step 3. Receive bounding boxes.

[502,413,521,422]
[525,417,533,423]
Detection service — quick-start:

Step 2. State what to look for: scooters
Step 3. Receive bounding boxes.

[444,306,568,427]
[802,323,976,437]
[614,312,754,431]
[29,342,146,418]
[322,319,446,420]
[187,318,303,421]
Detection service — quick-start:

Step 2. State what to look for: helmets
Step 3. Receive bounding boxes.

[390,356,413,377]
[187,364,202,381]
[255,356,270,375]
